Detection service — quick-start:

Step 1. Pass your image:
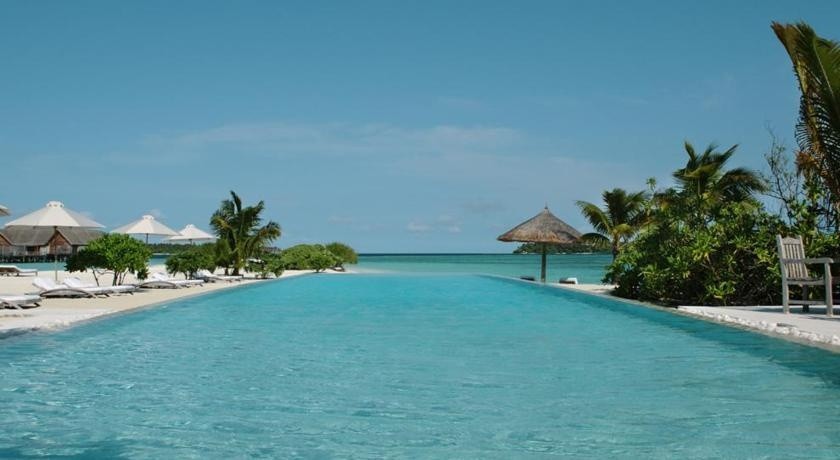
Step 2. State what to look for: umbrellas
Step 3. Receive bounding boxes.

[497,206,588,283]
[0,200,221,282]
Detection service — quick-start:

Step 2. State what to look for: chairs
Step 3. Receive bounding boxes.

[777,234,840,318]
[0,265,39,277]
[0,295,42,310]
[32,277,139,299]
[136,269,243,290]
